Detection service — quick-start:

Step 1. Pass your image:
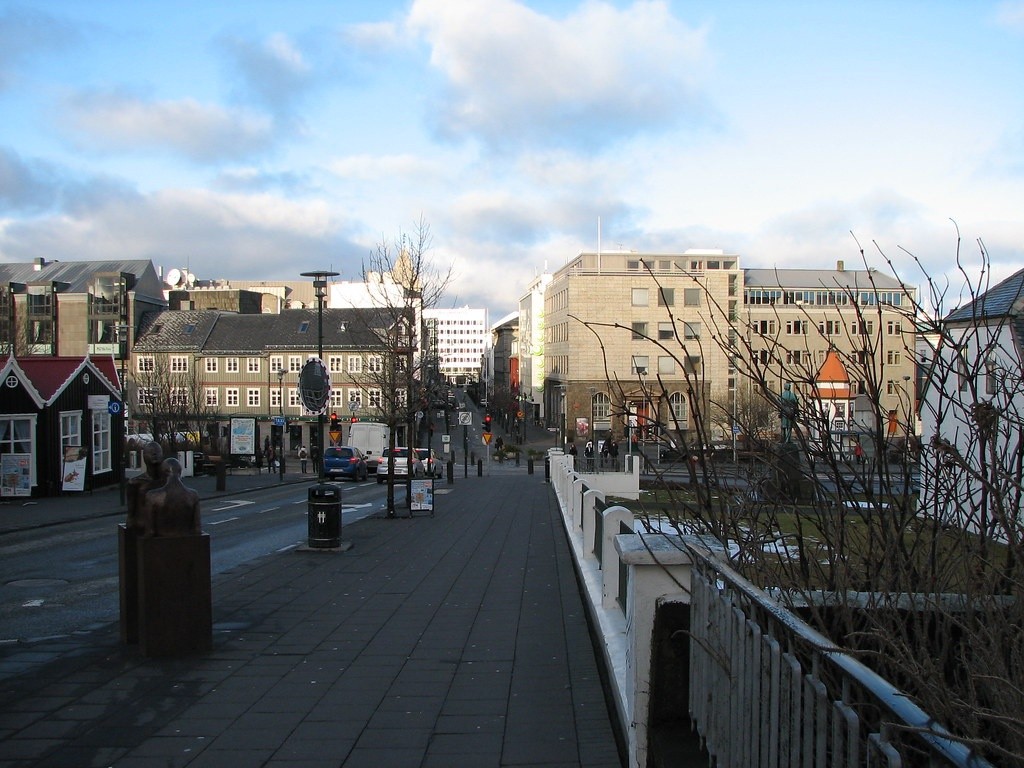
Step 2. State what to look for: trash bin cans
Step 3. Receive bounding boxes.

[309,485,342,548]
[193,452,205,476]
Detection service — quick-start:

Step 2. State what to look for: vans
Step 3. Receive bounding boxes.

[348,423,397,474]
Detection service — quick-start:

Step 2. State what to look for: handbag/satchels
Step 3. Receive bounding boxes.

[495,444,497,448]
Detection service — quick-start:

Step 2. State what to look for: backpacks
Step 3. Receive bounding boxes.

[300,450,306,458]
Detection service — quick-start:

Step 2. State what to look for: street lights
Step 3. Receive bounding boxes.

[741,341,751,441]
[278,369,288,480]
[301,271,340,494]
[729,388,737,462]
[561,391,566,451]
[642,371,648,475]
[903,376,910,463]
[624,400,633,455]
[109,324,137,504]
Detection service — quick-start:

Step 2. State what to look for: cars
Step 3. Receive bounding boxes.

[447,390,456,410]
[480,398,490,406]
[323,446,369,483]
[432,400,455,411]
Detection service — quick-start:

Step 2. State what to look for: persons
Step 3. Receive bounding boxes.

[855,443,862,464]
[778,383,798,443]
[605,429,612,445]
[568,439,619,470]
[496,435,503,450]
[262,435,278,474]
[298,445,319,474]
[126,442,201,537]
[430,423,435,437]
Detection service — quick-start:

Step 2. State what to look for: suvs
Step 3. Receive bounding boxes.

[376,448,425,484]
[416,448,444,479]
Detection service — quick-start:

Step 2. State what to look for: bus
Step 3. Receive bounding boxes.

[126,431,201,451]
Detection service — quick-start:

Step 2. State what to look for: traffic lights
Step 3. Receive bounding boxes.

[351,417,357,423]
[482,414,491,432]
[330,413,337,431]
